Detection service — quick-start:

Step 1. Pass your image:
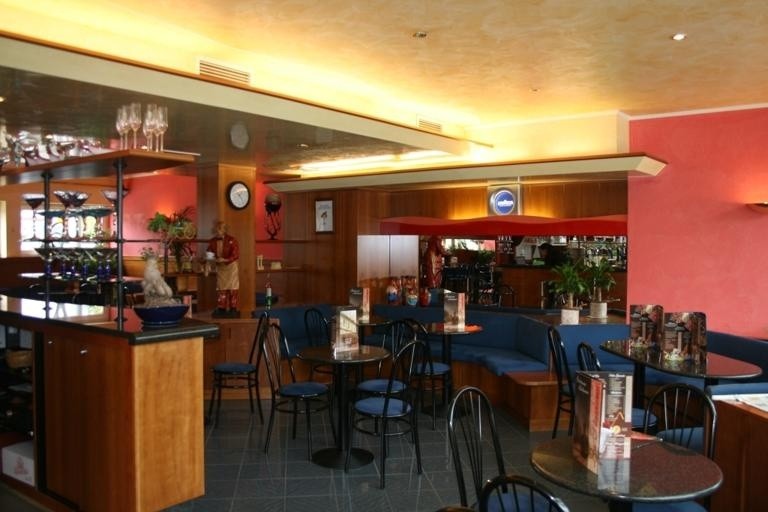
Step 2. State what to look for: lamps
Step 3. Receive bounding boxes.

[745,202,768,214]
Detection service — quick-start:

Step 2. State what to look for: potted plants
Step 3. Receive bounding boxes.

[148,212,197,272]
[547,259,591,323]
[580,255,617,318]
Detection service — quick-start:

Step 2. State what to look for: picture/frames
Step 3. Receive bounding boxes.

[314,197,337,234]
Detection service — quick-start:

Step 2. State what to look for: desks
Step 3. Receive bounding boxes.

[17,271,143,306]
[598,342,763,433]
[411,320,485,420]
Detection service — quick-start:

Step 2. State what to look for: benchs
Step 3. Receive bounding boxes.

[550,320,768,459]
[251,302,552,407]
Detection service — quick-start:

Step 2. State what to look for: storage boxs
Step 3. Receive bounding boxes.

[1,441,37,487]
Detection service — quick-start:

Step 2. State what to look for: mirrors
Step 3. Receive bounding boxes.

[387,234,627,323]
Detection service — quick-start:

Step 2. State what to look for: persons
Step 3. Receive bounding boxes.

[540,242,552,257]
[200,220,240,318]
[640,312,657,347]
[675,318,693,356]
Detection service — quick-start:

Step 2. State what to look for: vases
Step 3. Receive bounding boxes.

[384,275,418,308]
[419,285,432,307]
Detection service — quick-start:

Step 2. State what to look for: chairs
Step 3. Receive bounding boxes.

[206,311,268,428]
[445,385,556,511]
[433,264,564,309]
[547,326,584,439]
[258,307,451,489]
[576,342,658,438]
[629,381,718,512]
[478,474,573,512]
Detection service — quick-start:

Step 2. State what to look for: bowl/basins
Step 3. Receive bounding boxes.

[132,305,191,326]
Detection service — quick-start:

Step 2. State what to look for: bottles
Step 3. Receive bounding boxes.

[385,276,432,308]
[264,271,272,310]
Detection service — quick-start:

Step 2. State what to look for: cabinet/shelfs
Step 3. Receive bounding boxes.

[43,323,205,512]
[255,238,307,274]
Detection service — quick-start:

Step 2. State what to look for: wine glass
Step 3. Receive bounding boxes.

[22,188,117,286]
[114,103,169,152]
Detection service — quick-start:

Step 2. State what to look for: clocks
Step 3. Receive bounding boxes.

[226,181,251,210]
